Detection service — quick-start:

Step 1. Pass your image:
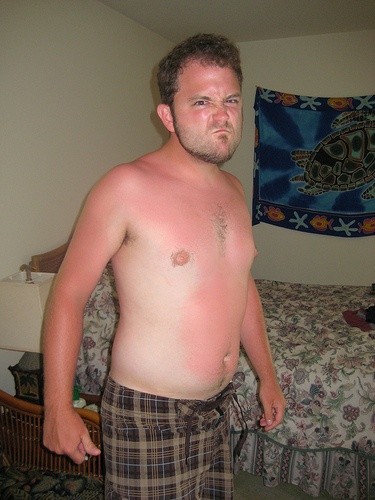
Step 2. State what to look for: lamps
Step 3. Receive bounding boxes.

[0,264,58,406]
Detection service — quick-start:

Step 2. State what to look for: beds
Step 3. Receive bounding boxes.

[31,240,375,500]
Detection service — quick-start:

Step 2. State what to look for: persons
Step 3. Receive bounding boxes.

[42,34,287,500]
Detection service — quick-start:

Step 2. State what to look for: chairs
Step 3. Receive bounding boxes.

[0,388,105,500]
